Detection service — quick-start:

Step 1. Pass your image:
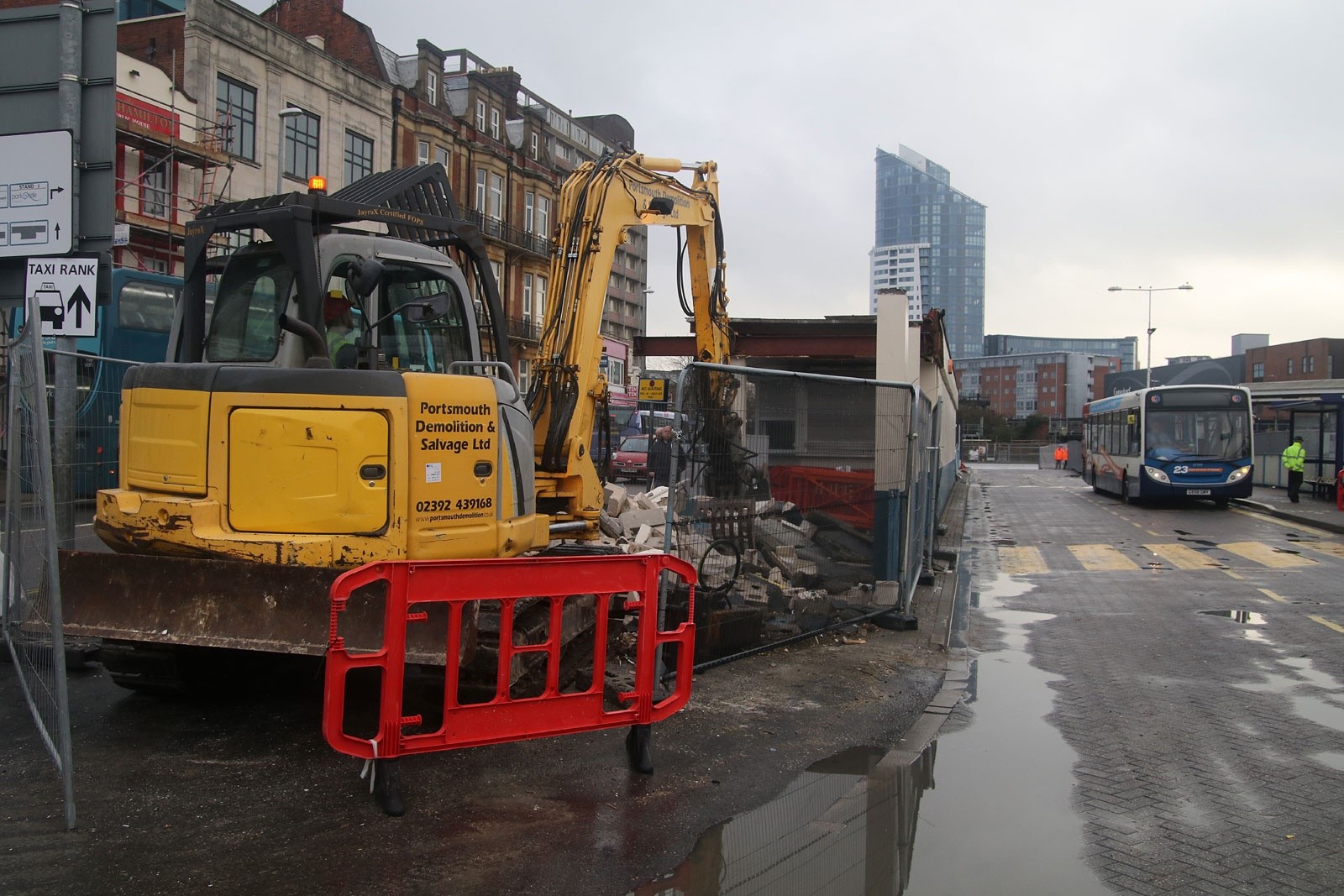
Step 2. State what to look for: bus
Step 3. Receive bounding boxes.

[1081,383,1261,508]
[591,409,708,463]
[3,263,435,508]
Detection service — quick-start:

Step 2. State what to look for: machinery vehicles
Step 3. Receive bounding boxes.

[87,147,773,571]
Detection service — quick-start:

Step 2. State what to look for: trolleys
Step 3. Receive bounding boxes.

[1304,475,1339,501]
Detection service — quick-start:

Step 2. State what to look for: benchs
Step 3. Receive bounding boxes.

[1304,476,1337,501]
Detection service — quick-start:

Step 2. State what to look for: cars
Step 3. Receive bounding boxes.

[606,433,658,482]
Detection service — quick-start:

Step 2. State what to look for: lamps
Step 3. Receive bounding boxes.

[130,70,140,76]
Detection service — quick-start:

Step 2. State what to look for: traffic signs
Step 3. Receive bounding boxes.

[21,257,98,337]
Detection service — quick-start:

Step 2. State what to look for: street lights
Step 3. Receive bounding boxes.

[1107,285,1195,388]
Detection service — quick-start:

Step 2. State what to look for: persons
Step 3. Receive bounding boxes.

[969,443,987,462]
[323,291,358,369]
[1146,419,1172,449]
[1282,436,1305,503]
[1054,444,1068,469]
[648,425,687,488]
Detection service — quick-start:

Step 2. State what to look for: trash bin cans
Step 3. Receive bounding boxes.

[1337,469,1344,512]
